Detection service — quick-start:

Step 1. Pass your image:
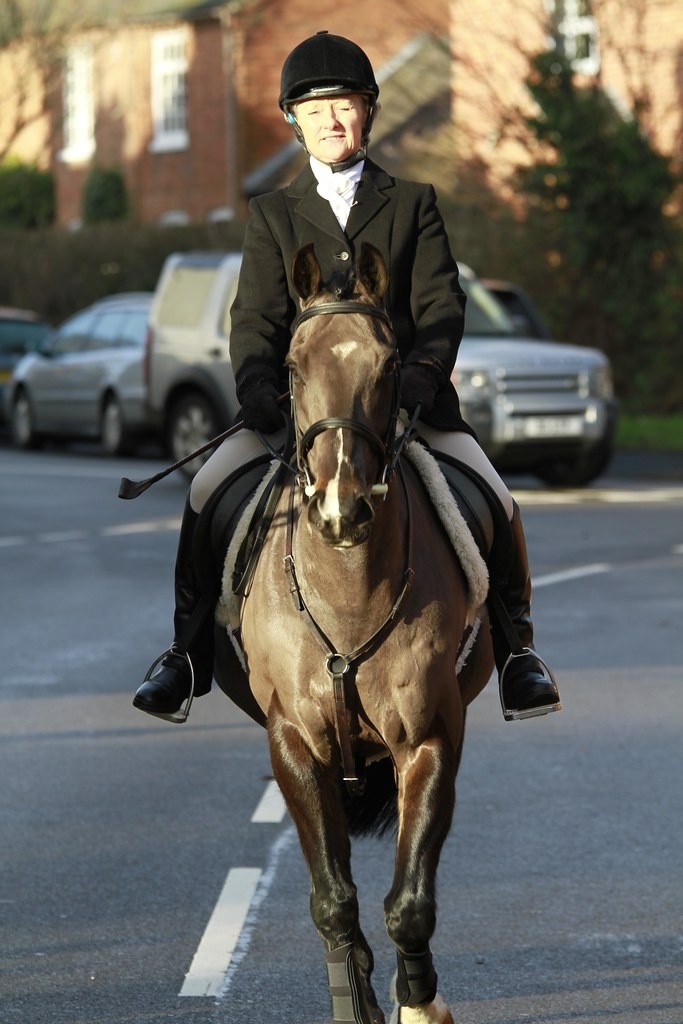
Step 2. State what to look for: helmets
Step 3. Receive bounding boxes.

[277,30,379,112]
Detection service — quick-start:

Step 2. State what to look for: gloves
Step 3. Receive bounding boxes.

[399,363,439,421]
[243,383,290,436]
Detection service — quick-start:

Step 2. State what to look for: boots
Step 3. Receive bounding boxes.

[132,488,222,716]
[478,497,560,711]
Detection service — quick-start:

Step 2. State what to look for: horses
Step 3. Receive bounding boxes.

[213,254,499,1023]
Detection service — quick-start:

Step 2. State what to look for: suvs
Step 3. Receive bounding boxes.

[144,250,618,488]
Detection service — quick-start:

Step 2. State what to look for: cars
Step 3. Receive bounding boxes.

[0,293,155,457]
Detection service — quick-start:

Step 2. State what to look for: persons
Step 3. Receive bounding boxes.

[131,29,567,719]
